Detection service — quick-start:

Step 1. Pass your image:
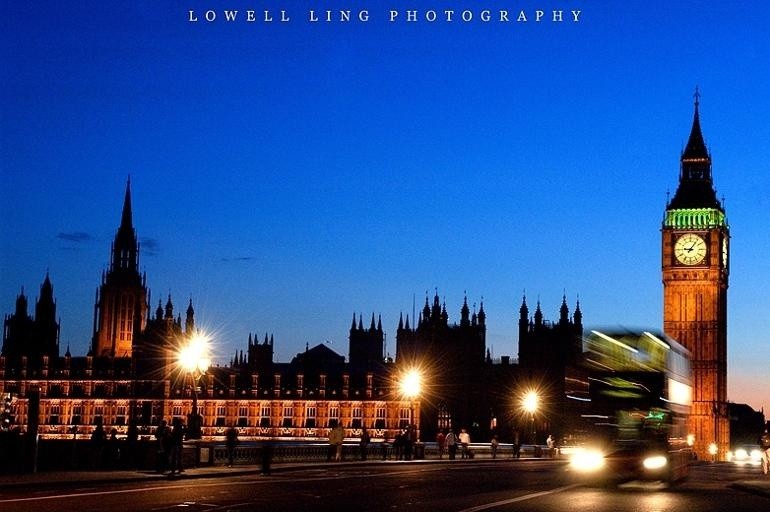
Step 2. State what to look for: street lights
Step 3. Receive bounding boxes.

[524,396,536,433]
[402,374,418,424]
[181,343,204,416]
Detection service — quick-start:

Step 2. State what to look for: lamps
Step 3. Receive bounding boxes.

[674,233,707,265]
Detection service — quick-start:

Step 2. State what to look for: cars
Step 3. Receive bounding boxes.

[736,443,762,461]
[755,419,770,475]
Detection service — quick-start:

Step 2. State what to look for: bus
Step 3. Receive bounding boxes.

[562,326,696,489]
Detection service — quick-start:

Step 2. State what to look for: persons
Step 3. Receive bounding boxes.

[327,422,346,462]
[436,429,470,460]
[224,421,239,468]
[491,435,499,459]
[512,438,522,458]
[360,428,370,459]
[392,426,416,460]
[546,435,555,458]
[90,417,188,474]
[260,465,271,476]
